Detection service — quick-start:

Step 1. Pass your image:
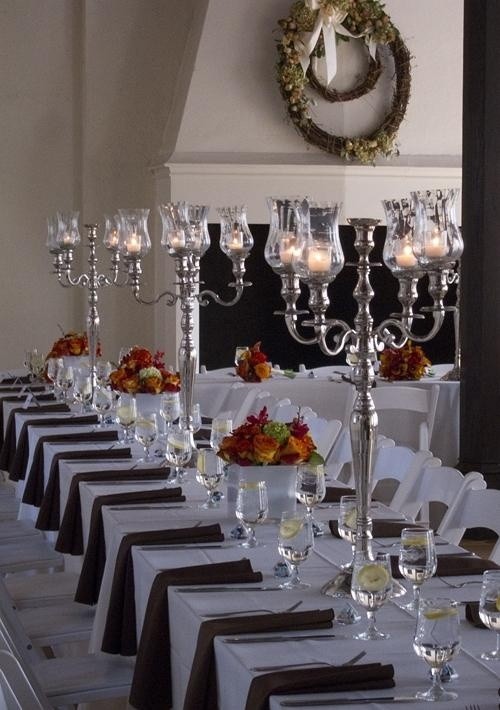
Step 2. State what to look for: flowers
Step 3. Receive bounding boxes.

[44,332,103,360]
[272,1,417,167]
[236,342,271,382]
[380,338,431,381]
[108,345,182,396]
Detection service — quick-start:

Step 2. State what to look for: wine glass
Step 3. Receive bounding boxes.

[277,509,312,591]
[31,353,47,385]
[118,346,132,364]
[196,447,223,510]
[89,385,112,430]
[235,347,249,367]
[166,432,193,485]
[295,463,326,536]
[96,360,109,387]
[478,569,500,661]
[48,358,64,394]
[351,550,392,641]
[135,413,160,466]
[160,391,179,435]
[399,527,438,609]
[56,366,74,403]
[191,404,202,446]
[338,495,359,570]
[235,479,268,549]
[23,349,37,377]
[210,419,232,476]
[73,376,93,415]
[116,397,136,446]
[413,599,463,701]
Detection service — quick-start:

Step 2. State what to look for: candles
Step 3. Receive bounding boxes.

[424,236,446,258]
[308,249,330,271]
[228,238,240,249]
[173,237,183,246]
[110,240,117,245]
[127,239,139,252]
[279,245,296,264]
[64,236,73,242]
[395,245,417,268]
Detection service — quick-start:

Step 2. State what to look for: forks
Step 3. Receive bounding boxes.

[439,576,483,588]
[465,704,480,710]
[200,601,303,617]
[373,540,449,547]
[251,650,366,670]
